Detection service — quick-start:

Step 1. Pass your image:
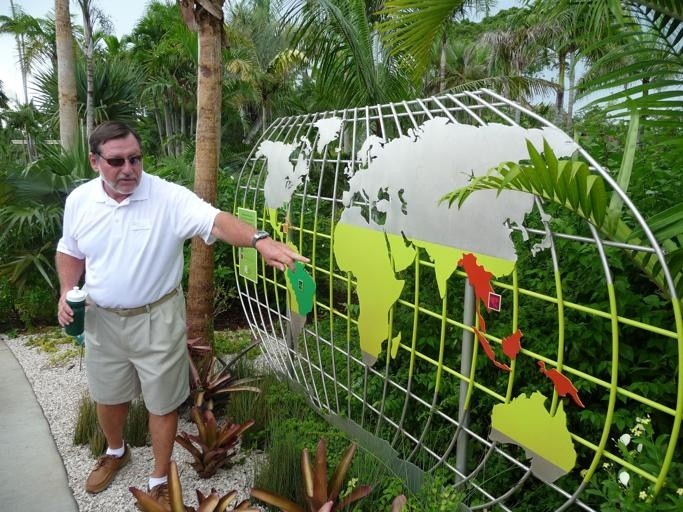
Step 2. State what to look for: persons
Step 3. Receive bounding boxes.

[54,119,310,508]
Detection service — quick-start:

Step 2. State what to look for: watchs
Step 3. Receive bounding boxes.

[250,230,268,250]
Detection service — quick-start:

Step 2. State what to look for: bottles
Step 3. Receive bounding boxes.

[64,286,85,336]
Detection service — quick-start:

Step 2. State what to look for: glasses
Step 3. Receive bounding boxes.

[98,151,143,166]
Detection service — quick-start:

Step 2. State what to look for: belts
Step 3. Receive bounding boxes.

[100,285,180,315]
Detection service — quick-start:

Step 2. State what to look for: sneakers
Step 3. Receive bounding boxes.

[85,441,130,494]
[147,482,171,511]
